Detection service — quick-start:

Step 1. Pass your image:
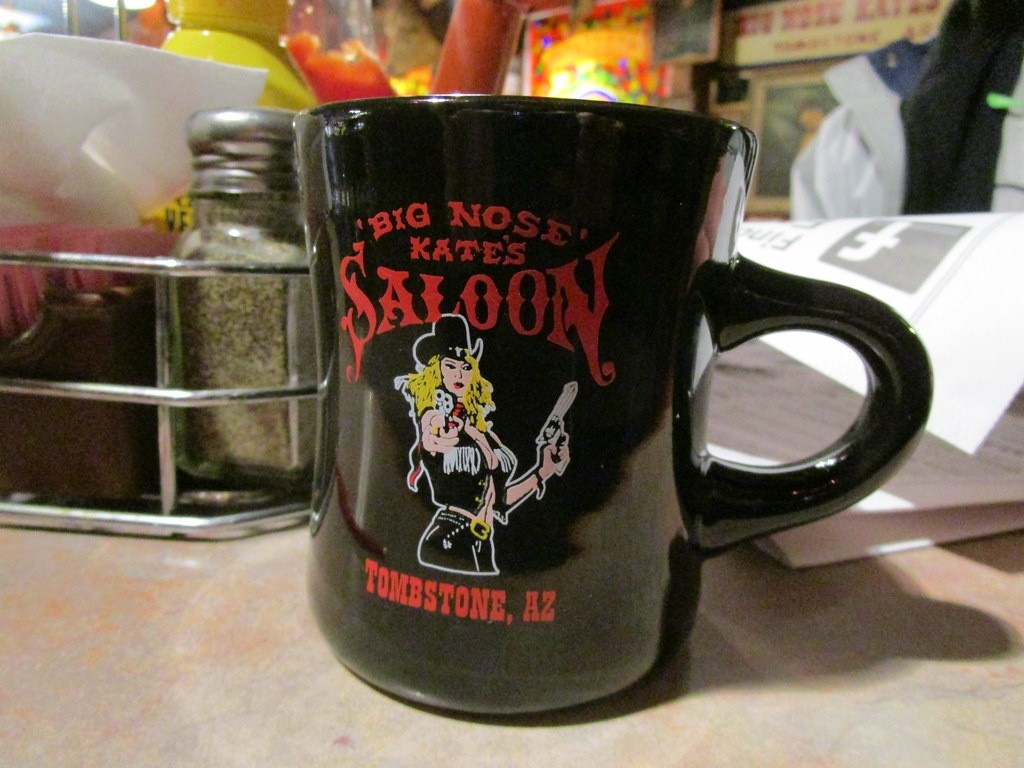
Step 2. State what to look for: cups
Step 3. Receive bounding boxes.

[290,88,931,714]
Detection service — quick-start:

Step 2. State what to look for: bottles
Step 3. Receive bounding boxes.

[162,105,337,487]
[151,1,321,125]
